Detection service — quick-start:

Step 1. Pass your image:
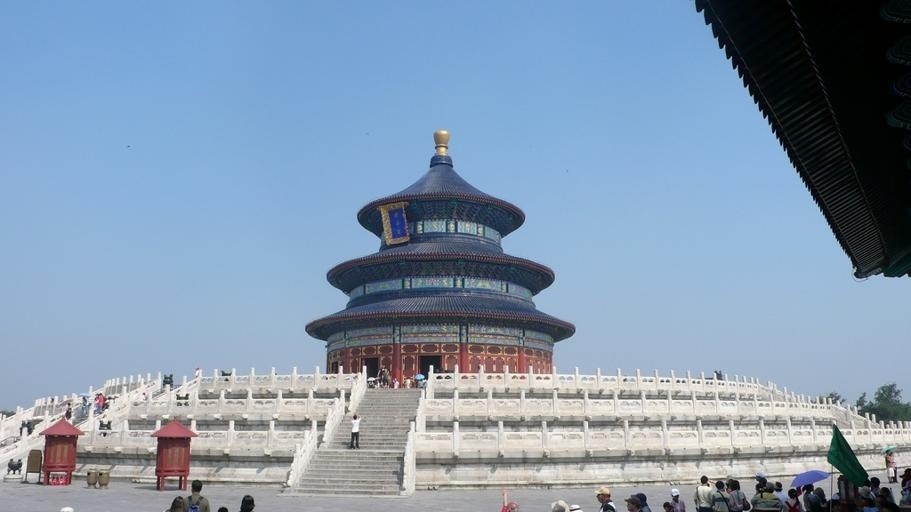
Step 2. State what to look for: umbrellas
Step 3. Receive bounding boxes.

[880,445,897,453]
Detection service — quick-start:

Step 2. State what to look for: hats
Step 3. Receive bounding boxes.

[672,488,680,496]
[632,493,647,501]
[625,497,642,505]
[552,500,570,512]
[596,486,610,495]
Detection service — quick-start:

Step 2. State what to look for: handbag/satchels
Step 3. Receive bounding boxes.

[743,500,750,511]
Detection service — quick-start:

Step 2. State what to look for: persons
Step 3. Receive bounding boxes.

[65,392,105,425]
[368,365,426,389]
[164,480,256,512]
[503,487,518,512]
[594,487,685,512]
[349,414,362,449]
[551,500,583,512]
[831,450,910,512]
[694,472,831,512]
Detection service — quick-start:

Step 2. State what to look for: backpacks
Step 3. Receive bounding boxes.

[188,495,204,512]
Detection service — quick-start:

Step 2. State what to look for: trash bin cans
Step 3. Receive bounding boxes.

[86,470,98,489]
[98,471,110,489]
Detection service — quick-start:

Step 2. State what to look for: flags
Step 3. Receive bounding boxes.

[827,425,869,487]
